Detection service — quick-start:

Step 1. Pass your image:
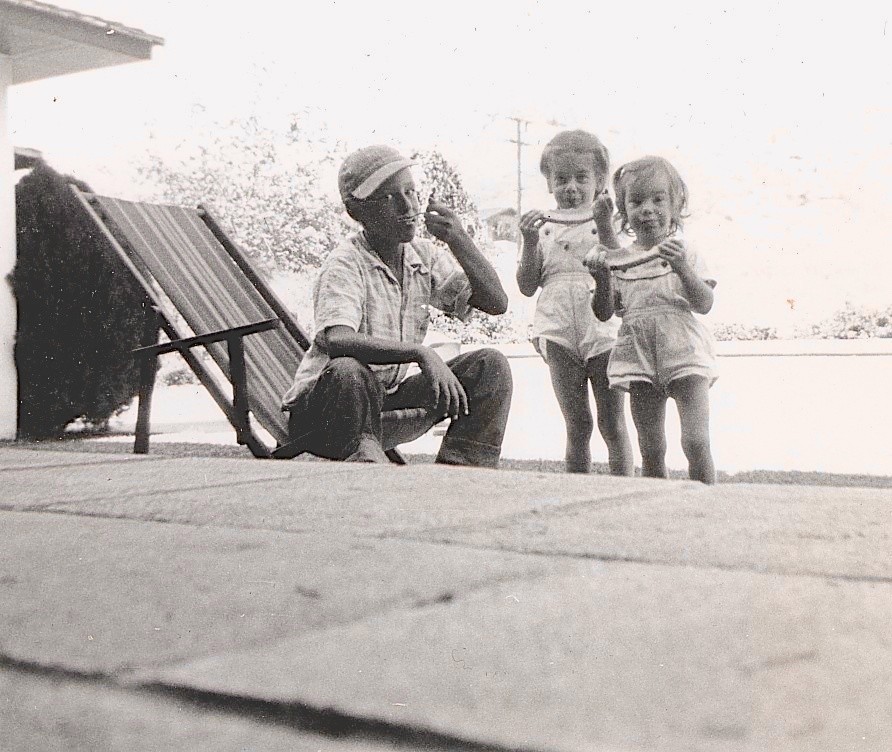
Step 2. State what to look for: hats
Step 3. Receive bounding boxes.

[337,145,419,204]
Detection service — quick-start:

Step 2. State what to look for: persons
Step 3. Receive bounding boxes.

[516,129,634,477]
[583,156,717,487]
[280,144,513,469]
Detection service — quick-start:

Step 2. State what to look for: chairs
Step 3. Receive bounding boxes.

[65,184,452,466]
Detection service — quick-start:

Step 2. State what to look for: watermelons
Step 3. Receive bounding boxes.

[540,201,596,224]
[588,244,663,270]
